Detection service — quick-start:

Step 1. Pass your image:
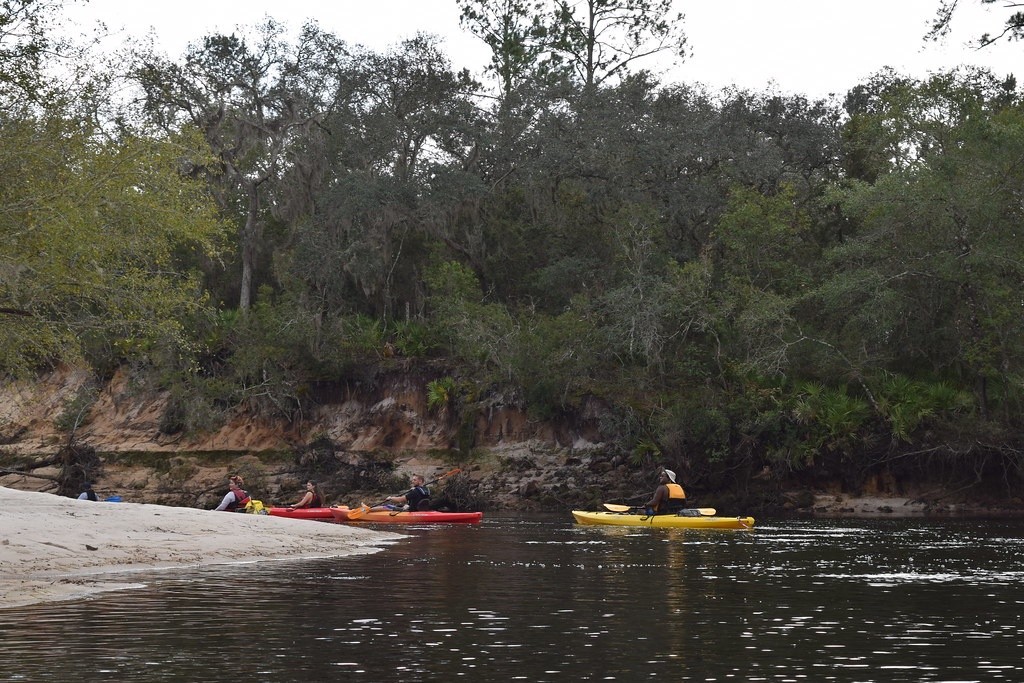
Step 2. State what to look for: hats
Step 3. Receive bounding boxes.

[665,470,677,483]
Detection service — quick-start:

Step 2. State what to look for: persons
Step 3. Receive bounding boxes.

[643,469,686,516]
[215,476,251,513]
[77,483,101,501]
[385,473,431,512]
[290,480,323,508]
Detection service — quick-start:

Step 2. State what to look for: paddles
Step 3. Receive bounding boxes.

[286,506,298,511]
[349,469,464,518]
[603,502,716,516]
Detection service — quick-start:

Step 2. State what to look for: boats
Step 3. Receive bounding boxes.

[328,507,485,521]
[570,509,756,532]
[237,505,348,519]
